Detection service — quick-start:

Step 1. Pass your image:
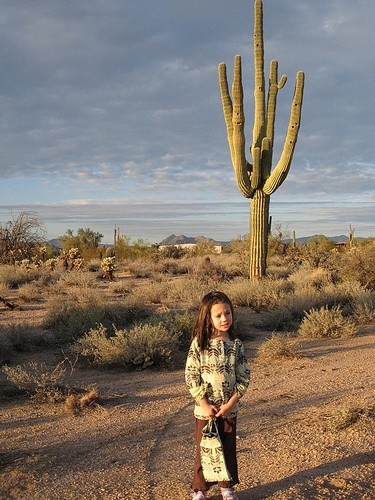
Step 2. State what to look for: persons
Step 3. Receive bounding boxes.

[184,292,250,500]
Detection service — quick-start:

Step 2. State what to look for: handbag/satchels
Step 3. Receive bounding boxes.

[199,416,232,482]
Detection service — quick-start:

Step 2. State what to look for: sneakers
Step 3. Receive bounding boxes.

[220,487,239,500]
[192,491,205,500]
